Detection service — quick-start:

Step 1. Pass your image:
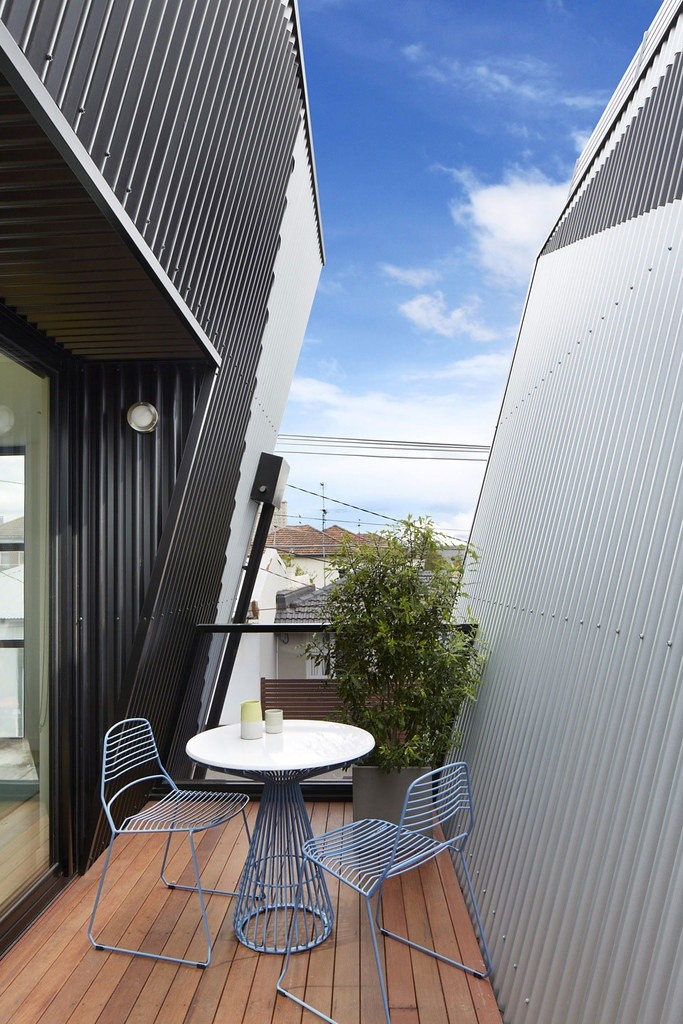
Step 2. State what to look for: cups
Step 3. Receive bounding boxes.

[265,709,283,734]
[240,700,263,740]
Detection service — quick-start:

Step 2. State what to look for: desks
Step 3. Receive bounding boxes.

[184,721,377,948]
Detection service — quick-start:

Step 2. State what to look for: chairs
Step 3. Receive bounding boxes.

[86,716,265,969]
[277,761,498,1024]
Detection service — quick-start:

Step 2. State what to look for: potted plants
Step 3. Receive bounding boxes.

[294,511,490,857]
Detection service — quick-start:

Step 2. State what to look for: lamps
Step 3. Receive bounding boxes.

[126,400,160,433]
